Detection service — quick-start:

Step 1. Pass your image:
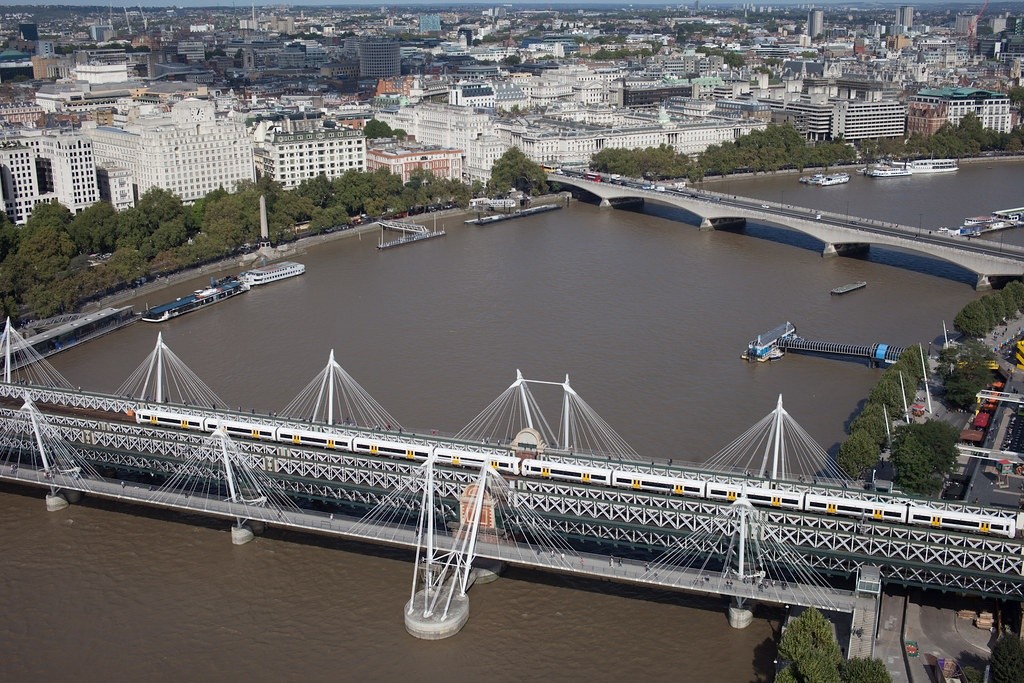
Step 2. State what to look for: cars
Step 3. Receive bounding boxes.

[324,199,457,234]
[538,163,700,200]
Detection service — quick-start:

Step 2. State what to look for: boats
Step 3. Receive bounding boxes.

[908,158,960,173]
[141,277,251,323]
[934,205,1024,237]
[376,228,446,251]
[871,167,913,177]
[798,172,826,184]
[242,260,307,287]
[741,320,796,363]
[463,203,563,227]
[830,280,867,296]
[820,171,850,186]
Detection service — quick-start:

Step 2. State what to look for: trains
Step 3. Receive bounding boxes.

[134,405,1017,548]
